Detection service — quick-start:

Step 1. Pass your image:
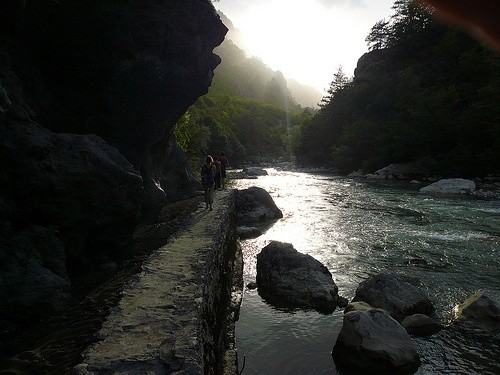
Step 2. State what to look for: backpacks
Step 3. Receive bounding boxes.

[203,164,215,186]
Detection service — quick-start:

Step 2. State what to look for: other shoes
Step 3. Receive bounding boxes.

[205,206,209,209]
[210,208,212,211]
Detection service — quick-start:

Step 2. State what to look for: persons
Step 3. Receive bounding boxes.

[201,156,217,212]
[213,154,222,190]
[219,151,230,190]
